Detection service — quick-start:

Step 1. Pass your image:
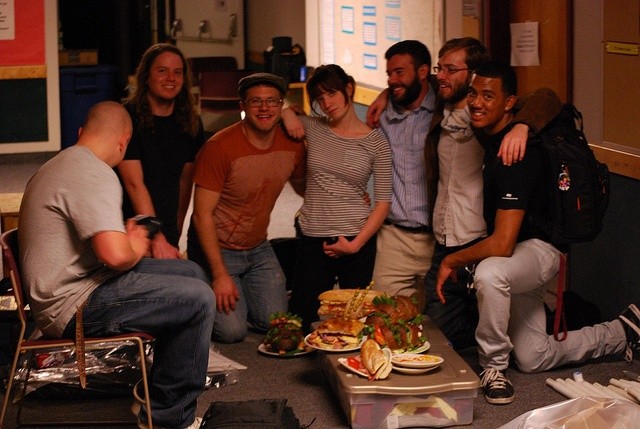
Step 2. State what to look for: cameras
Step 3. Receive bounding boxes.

[129,213,166,235]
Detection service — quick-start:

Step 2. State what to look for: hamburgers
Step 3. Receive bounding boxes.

[307,316,365,347]
[317,289,386,319]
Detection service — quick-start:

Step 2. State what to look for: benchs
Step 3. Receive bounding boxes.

[199,70,267,118]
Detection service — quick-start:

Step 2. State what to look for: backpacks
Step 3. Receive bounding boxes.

[200,398,316,429]
[536,101,610,242]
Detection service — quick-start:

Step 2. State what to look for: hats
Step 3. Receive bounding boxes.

[238,73,289,97]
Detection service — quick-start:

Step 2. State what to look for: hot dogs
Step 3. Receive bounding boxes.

[361,339,393,380]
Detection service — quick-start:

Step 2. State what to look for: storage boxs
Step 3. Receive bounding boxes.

[312,316,479,428]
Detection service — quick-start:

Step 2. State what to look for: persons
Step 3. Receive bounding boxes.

[120,42,205,258]
[17,101,206,428]
[285,63,392,321]
[437,60,640,407]
[362,40,445,296]
[193,73,371,343]
[367,37,601,348]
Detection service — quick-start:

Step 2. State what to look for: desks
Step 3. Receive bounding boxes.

[0,193,26,233]
[287,82,312,118]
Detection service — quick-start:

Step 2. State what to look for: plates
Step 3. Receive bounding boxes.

[338,346,393,378]
[389,364,441,374]
[410,341,431,353]
[258,343,310,357]
[392,353,445,367]
[304,334,367,352]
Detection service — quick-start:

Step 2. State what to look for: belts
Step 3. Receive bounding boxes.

[386,222,432,233]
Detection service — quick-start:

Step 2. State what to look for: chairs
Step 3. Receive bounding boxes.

[187,56,237,105]
[0,228,154,429]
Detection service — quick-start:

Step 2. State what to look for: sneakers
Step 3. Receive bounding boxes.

[619,303,640,362]
[480,366,515,404]
[137,402,204,429]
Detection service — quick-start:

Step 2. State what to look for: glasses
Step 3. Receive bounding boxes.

[247,97,282,107]
[433,65,472,74]
